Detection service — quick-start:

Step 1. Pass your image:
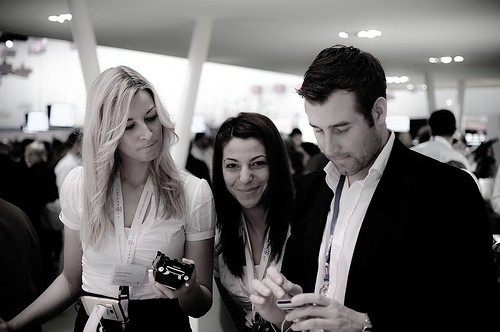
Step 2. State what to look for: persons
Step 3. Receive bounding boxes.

[212,112,297,332]
[0,129,85,332]
[393,109,500,286]
[0,66,218,332]
[186,120,330,193]
[250,43,500,332]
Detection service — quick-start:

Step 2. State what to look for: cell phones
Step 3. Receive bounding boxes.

[276,300,312,313]
[81,295,126,321]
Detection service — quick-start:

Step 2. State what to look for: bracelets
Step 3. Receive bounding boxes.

[362,315,372,332]
